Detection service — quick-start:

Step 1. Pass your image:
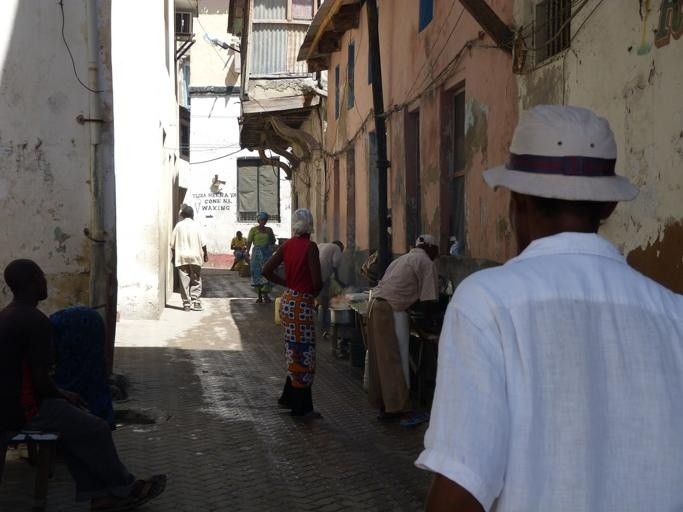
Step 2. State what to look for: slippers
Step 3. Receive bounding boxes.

[118,474,167,512]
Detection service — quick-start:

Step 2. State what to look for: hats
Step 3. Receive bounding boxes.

[479,104,640,202]
[256,212,268,222]
[415,234,438,247]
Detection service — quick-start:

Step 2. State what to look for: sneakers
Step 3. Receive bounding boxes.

[183,300,191,311]
[194,303,203,310]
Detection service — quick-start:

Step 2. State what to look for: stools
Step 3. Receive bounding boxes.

[0,431,61,512]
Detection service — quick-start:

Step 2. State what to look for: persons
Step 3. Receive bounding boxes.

[0,259,167,510]
[230,231,251,271]
[170,206,209,311]
[246,212,276,303]
[367,234,440,426]
[316,240,348,337]
[414,104,683,512]
[261,207,322,418]
